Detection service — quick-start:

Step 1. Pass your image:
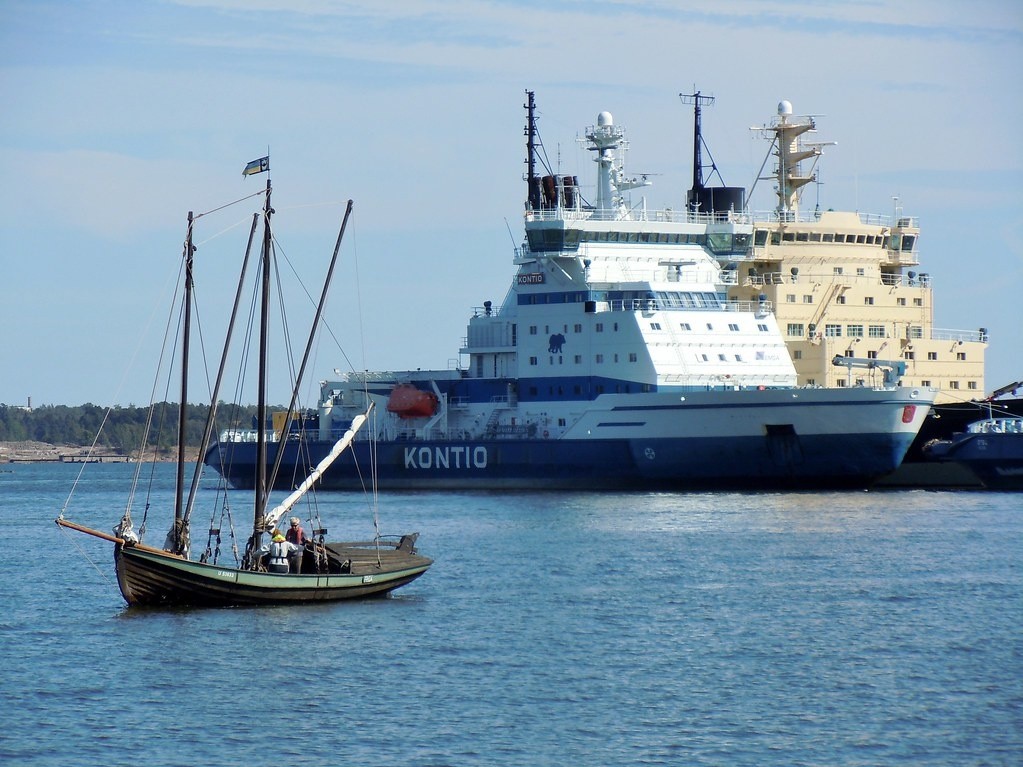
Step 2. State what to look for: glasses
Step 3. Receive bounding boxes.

[291,524,298,527]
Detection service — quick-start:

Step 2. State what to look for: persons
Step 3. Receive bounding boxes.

[378,428,384,441]
[286,516,311,574]
[412,429,417,440]
[492,425,497,438]
[251,533,307,575]
[543,430,549,440]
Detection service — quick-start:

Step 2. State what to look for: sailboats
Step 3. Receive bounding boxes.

[50,146,436,610]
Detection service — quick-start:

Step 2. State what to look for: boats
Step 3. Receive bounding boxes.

[206,83,942,491]
[921,401,1023,489]
[727,97,1023,490]
[385,383,438,418]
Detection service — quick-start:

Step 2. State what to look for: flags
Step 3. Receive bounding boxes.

[243,157,269,177]
